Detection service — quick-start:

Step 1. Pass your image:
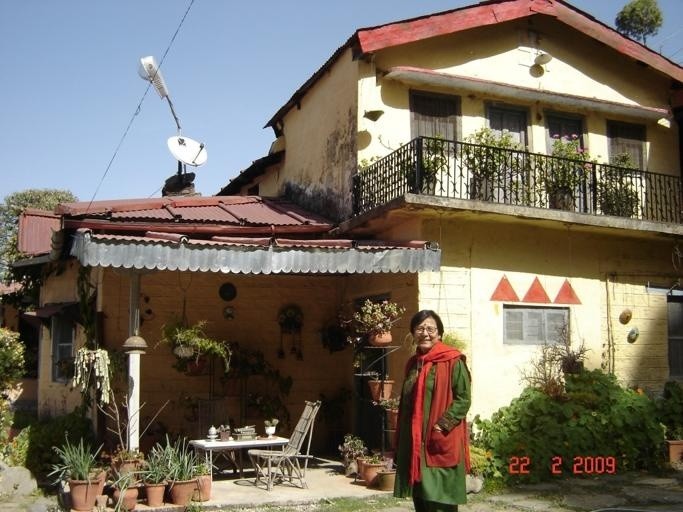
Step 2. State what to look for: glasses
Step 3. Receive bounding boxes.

[414,324,437,333]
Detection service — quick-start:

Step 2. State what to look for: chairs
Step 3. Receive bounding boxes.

[248,400,321,493]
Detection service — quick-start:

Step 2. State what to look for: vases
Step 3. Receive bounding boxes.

[368,330,393,347]
[368,380,396,402]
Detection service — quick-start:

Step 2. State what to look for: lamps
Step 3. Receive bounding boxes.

[535,39,552,66]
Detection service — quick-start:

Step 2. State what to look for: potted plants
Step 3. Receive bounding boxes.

[161,320,233,372]
[264,418,279,439]
[46,430,212,511]
[406,132,639,217]
[338,433,397,491]
[72,346,112,404]
[378,400,400,449]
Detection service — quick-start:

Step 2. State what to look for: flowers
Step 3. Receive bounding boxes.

[351,298,407,335]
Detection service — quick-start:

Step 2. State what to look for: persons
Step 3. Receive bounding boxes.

[388,309,473,512]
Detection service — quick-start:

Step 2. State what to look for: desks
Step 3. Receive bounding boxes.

[189,434,289,479]
